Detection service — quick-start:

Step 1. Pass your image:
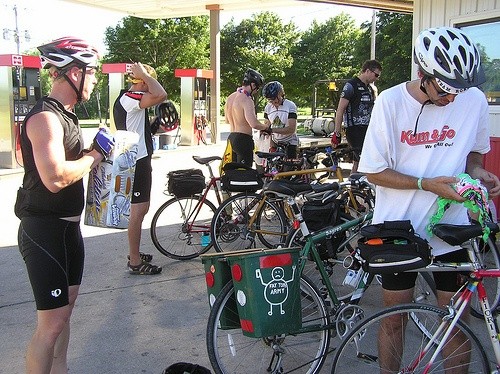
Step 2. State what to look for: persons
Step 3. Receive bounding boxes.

[357,27,500,374]
[14,35,115,374]
[113,62,168,276]
[260,82,298,210]
[331,60,383,212]
[219,68,272,225]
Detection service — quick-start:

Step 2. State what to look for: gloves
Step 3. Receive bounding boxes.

[93,129,115,157]
[260,127,273,137]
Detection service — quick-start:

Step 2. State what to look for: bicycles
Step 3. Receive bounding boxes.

[329,222,500,374]
[148,141,499,374]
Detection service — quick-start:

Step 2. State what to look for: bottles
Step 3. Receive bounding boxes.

[341,250,364,290]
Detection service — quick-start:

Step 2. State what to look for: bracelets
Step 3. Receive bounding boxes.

[417,177,425,191]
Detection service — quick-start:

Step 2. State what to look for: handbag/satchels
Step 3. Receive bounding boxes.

[221,163,264,192]
[301,198,345,262]
[168,169,206,197]
[357,220,433,274]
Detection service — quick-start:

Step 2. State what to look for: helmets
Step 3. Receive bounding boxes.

[414,27,486,94]
[155,101,178,131]
[37,36,99,71]
[262,81,282,100]
[244,68,265,87]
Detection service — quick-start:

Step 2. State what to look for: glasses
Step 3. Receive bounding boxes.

[369,69,381,78]
[429,79,448,97]
[255,83,259,92]
[79,68,95,75]
[267,96,278,103]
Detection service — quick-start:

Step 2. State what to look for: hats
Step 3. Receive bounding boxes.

[125,64,158,84]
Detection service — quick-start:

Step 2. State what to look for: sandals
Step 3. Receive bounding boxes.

[127,252,153,266]
[129,261,162,275]
